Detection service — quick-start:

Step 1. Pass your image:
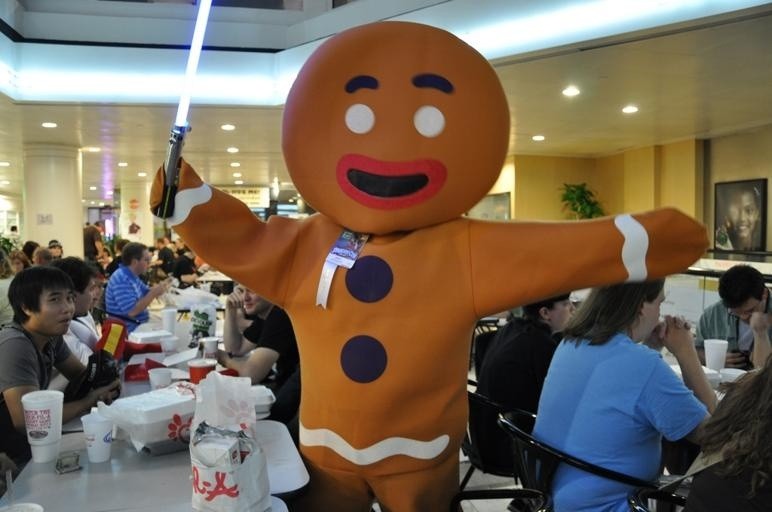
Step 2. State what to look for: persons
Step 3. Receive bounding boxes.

[696,266,772,369]
[1,219,210,467]
[473,293,577,512]
[523,277,719,512]
[215,284,301,420]
[680,354,772,512]
[715,184,762,251]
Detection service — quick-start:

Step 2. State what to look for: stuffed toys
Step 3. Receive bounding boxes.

[149,21,709,512]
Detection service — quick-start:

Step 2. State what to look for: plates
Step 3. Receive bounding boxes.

[255,419,311,494]
[270,496,289,512]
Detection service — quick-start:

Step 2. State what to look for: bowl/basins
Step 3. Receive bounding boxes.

[721,367,747,380]
[161,338,181,353]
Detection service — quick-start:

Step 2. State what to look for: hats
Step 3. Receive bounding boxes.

[49,240,62,248]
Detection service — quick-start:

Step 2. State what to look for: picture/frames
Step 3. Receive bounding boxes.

[714,179,767,261]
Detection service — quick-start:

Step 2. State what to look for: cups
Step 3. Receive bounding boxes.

[21,391,64,466]
[188,359,217,384]
[704,339,728,370]
[80,415,111,465]
[200,282,210,293]
[200,337,218,358]
[148,369,171,388]
[163,309,176,334]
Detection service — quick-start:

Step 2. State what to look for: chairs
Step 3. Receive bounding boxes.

[628,488,685,512]
[450,489,547,512]
[460,379,531,489]
[498,409,659,511]
